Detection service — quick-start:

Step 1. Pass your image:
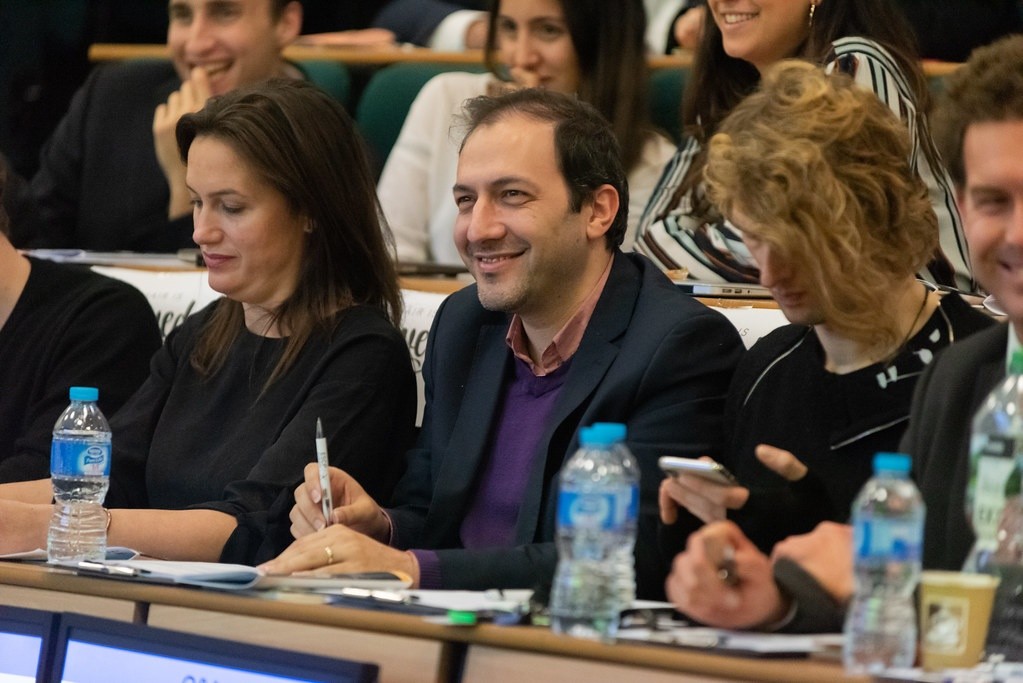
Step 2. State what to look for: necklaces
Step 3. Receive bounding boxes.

[883,284,928,369]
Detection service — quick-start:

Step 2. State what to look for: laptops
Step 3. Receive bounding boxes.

[676,282,776,300]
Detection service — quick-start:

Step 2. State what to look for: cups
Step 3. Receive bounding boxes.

[918,570,1002,672]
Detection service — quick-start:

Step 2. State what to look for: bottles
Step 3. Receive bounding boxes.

[961,347,1023,683]
[549,427,629,647]
[840,453,925,674]
[591,422,641,604]
[45,386,111,565]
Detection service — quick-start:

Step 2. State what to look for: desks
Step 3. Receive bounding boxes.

[0,550,865,683]
[18,250,795,312]
[81,29,982,78]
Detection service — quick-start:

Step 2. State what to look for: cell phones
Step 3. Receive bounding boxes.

[659,455,740,488]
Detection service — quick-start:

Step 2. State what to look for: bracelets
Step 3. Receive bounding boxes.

[104,508,111,528]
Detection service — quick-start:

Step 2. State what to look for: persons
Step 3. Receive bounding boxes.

[531,56,1000,603]
[0,205,163,485]
[381,0,706,51]
[375,0,678,259]
[665,30,1023,664]
[257,87,745,589]
[633,0,983,293]
[0,86,417,567]
[0,0,304,252]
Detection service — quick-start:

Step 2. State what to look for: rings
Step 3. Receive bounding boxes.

[326,546,332,564]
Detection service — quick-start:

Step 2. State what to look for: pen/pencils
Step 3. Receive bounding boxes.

[316,417,333,528]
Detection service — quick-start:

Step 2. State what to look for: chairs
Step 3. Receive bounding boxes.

[69,58,806,391]
